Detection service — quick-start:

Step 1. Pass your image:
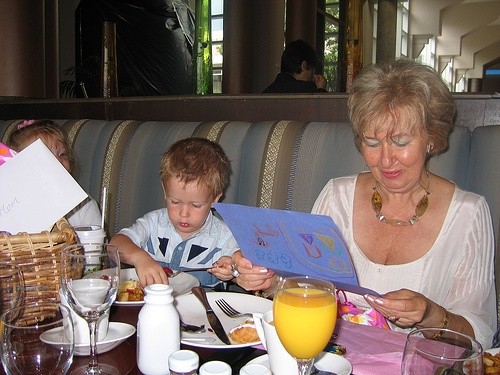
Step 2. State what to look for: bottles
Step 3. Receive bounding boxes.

[167,350,200,375]
[198,361,233,375]
[238,363,272,375]
[136,283,181,375]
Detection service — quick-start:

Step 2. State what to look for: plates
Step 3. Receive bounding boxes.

[40,321,137,356]
[463,347,500,375]
[171,291,275,349]
[82,267,202,306]
[245,351,353,375]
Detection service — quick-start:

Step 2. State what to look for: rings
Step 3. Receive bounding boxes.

[388,316,399,323]
[230,265,238,277]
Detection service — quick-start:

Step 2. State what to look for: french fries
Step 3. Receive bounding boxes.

[463,351,500,375]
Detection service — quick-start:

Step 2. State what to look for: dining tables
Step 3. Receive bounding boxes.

[0,289,469,375]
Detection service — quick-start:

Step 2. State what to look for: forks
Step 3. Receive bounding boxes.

[166,264,220,278]
[216,298,254,318]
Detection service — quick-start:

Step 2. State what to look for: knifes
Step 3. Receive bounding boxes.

[190,286,231,345]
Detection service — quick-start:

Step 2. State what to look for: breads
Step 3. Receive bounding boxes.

[231,320,260,343]
[114,279,144,301]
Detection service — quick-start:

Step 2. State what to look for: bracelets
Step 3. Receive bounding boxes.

[429,306,449,340]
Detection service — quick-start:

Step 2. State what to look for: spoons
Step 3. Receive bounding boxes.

[172,294,206,334]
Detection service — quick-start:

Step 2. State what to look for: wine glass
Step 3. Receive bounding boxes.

[59,243,121,375]
[272,276,338,375]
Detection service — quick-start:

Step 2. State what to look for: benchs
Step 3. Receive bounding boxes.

[0,118,500,350]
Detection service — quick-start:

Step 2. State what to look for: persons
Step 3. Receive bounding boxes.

[0,118,105,277]
[231,57,498,353]
[260,39,331,93]
[106,137,240,293]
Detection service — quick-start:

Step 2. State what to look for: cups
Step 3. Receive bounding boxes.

[253,311,298,375]
[60,278,110,345]
[73,225,109,277]
[401,328,485,375]
[0,261,26,325]
[0,301,75,375]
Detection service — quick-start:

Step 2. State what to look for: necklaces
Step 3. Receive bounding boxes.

[371,168,430,227]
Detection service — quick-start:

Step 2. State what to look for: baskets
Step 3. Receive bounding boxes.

[0,217,85,327]
